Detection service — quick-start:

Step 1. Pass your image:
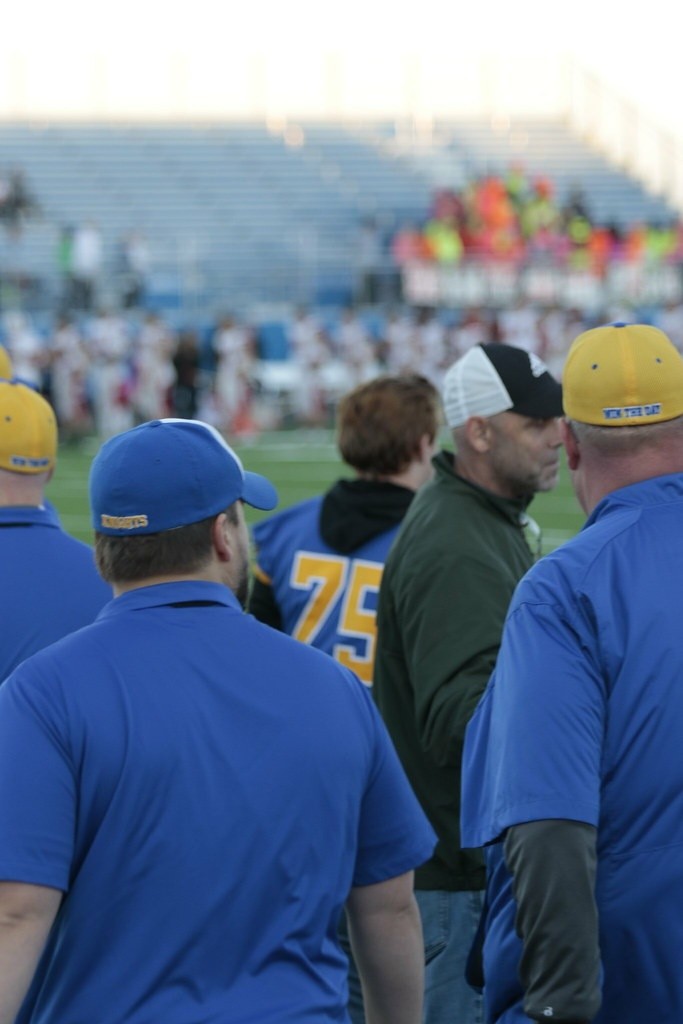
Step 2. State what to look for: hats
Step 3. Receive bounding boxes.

[441,342,565,429]
[88,417,275,536]
[0,380,58,472]
[560,321,683,426]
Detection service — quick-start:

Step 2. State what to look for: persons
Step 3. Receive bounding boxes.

[341,341,566,1023]
[0,288,683,434]
[457,326,683,1024]
[0,379,115,685]
[254,372,444,696]
[0,417,441,1024]
